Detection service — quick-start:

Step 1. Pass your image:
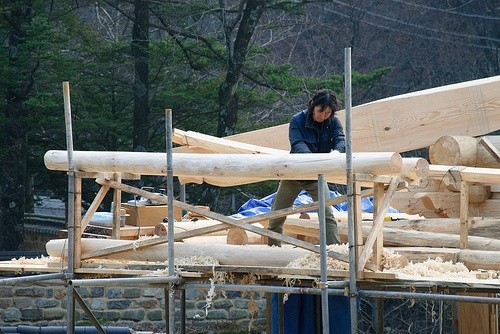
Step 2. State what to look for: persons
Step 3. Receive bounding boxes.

[268,89,346,246]
